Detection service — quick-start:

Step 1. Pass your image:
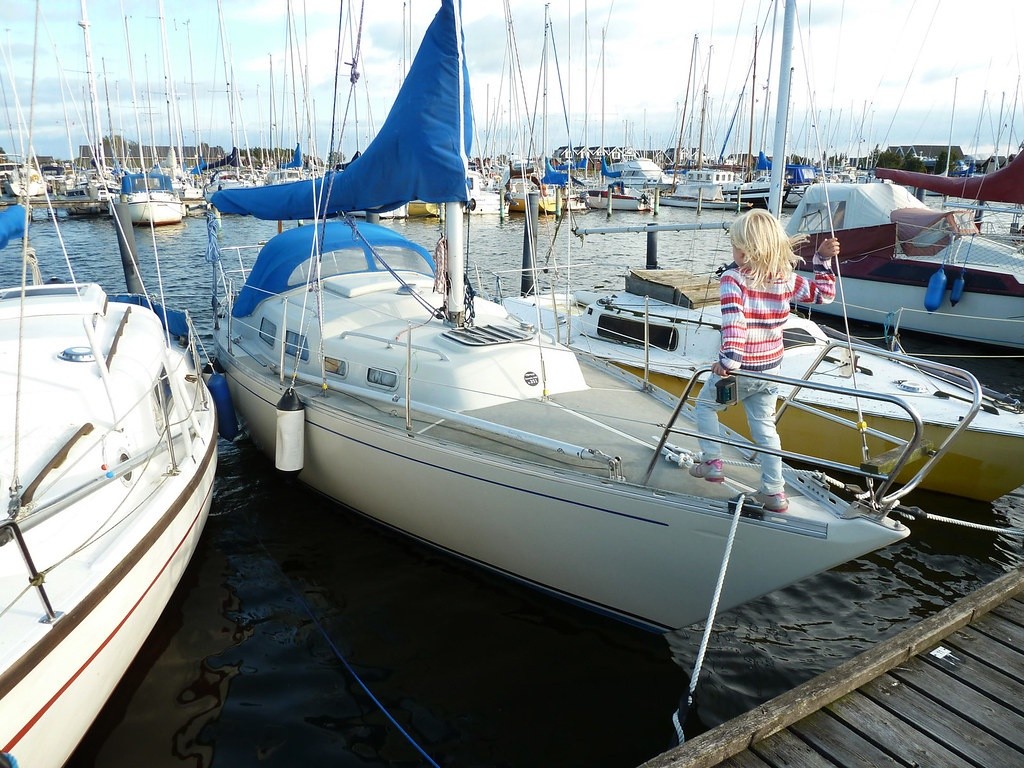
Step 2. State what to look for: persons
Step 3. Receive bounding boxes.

[689,209,840,513]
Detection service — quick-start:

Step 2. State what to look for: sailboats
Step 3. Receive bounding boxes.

[1,0,1021,768]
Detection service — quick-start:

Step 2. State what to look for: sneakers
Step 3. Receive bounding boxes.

[738,490,788,513]
[689,459,725,482]
